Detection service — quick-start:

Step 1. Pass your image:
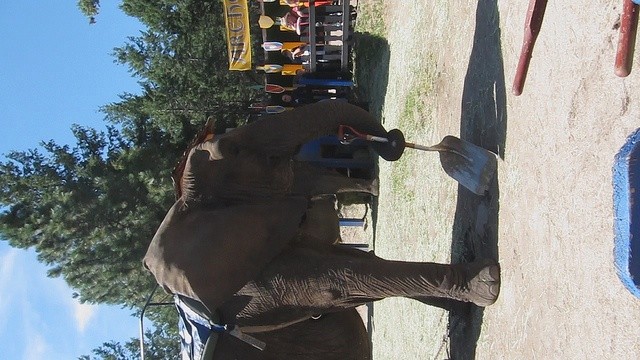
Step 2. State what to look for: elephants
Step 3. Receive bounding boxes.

[142,99,499,360]
